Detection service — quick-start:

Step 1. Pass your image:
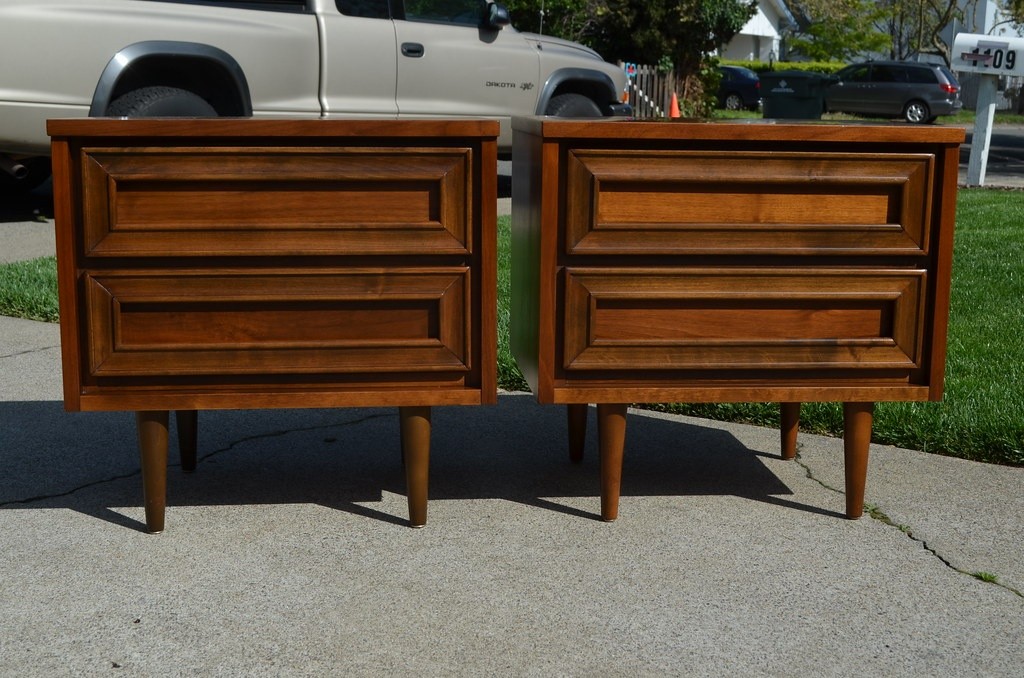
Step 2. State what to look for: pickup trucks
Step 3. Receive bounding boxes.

[1,1,630,191]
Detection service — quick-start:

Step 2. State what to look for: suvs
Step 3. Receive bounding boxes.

[824,59,964,125]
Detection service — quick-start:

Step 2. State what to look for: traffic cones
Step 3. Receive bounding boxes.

[668,92,680,118]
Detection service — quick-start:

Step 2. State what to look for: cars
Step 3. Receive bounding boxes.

[712,65,761,112]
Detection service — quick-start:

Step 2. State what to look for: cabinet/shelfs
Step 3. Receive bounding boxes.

[47,116,500,533]
[508,114,967,525]
